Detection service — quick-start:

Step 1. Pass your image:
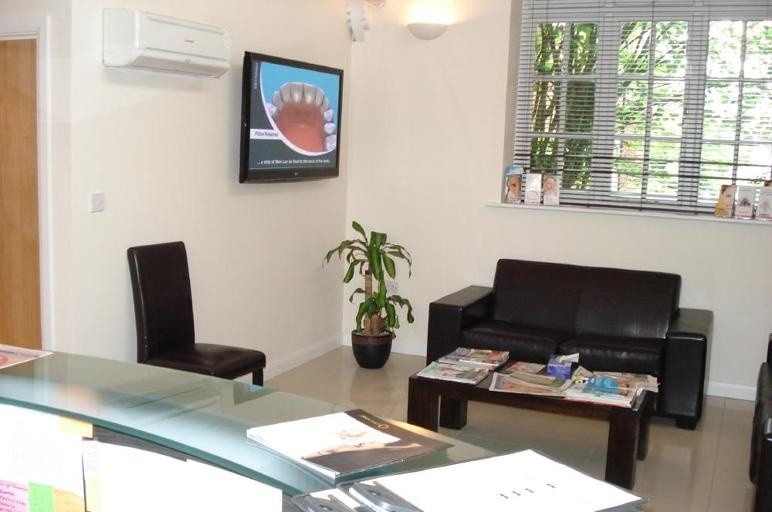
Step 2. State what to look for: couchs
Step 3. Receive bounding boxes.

[427,258,713,429]
[749,333,772,512]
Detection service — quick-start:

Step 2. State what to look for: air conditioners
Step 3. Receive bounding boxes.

[102,8,231,79]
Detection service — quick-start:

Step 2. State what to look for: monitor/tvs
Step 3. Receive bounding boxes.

[238,50,344,183]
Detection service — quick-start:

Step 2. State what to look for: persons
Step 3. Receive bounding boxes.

[721,185,737,213]
[544,177,555,195]
[507,176,522,202]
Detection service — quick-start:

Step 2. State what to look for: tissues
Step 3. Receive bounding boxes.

[546,353,581,379]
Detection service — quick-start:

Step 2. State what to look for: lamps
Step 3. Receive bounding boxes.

[406,8,448,40]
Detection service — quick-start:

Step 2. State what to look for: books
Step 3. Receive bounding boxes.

[417,343,660,408]
[345,448,652,511]
[247,403,455,481]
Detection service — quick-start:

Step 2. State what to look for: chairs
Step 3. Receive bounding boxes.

[127,242,265,386]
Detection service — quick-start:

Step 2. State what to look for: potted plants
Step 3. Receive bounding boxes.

[325,221,414,369]
